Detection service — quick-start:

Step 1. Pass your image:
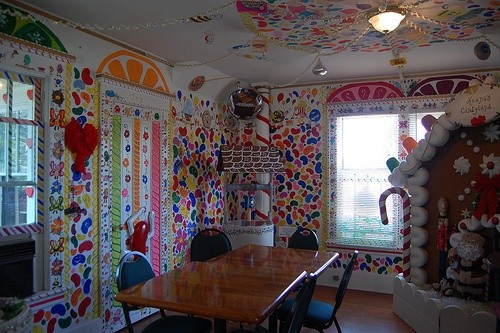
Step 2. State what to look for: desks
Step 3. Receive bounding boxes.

[223,220,275,247]
[114,243,340,324]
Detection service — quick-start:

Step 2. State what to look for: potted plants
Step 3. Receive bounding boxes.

[0,295,31,333]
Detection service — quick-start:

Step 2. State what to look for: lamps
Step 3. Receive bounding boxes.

[367,0,406,35]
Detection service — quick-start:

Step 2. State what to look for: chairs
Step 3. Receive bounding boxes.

[287,228,319,250]
[229,250,358,333]
[190,229,233,261]
[116,250,212,333]
